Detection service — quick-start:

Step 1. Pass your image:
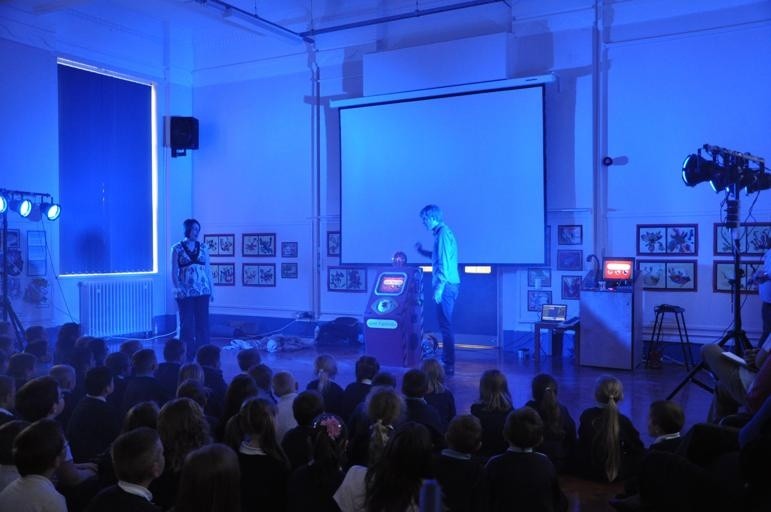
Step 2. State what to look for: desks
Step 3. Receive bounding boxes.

[535,317,580,367]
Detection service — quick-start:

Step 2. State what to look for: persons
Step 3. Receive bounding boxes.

[0,333,770,512]
[414,204,460,375]
[753,236,771,347]
[170,218,213,363]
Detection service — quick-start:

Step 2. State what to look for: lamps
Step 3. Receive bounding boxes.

[0,188,61,222]
[681,144,771,194]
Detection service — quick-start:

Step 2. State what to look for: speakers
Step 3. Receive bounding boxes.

[174,116,199,150]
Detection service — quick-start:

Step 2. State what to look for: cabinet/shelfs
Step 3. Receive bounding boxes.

[579,268,642,371]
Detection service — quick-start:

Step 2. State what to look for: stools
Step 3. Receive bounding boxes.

[644,304,696,371]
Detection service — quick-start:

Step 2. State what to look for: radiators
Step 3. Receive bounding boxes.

[78,278,155,343]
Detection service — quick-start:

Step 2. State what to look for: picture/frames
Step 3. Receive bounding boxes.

[203,233,298,288]
[527,225,583,324]
[0,229,20,247]
[635,223,771,293]
[327,230,341,256]
[327,266,367,293]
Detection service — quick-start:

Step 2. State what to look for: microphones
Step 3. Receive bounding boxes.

[587,255,600,288]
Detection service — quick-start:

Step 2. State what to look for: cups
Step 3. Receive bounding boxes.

[599,281,606,291]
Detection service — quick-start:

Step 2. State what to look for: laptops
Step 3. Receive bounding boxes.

[536,304,567,325]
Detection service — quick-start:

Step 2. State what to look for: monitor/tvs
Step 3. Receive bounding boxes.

[601,258,635,287]
